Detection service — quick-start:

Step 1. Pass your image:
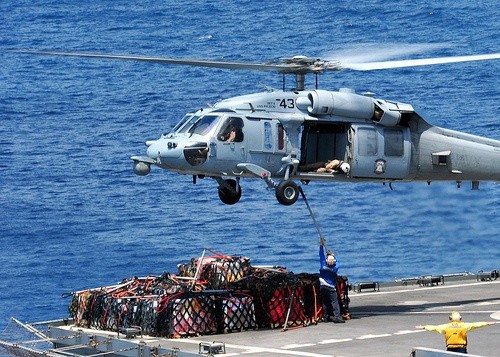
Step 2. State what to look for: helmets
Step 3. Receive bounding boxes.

[340,162,351,173]
[326,255,336,266]
[449,311,462,322]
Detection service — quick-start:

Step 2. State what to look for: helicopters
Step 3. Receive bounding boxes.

[0,44,500,205]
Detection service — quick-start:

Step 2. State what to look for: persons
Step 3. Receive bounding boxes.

[226,118,243,142]
[298,159,350,175]
[415,311,496,354]
[319,237,345,324]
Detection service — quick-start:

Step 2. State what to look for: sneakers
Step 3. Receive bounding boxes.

[330,315,345,322]
